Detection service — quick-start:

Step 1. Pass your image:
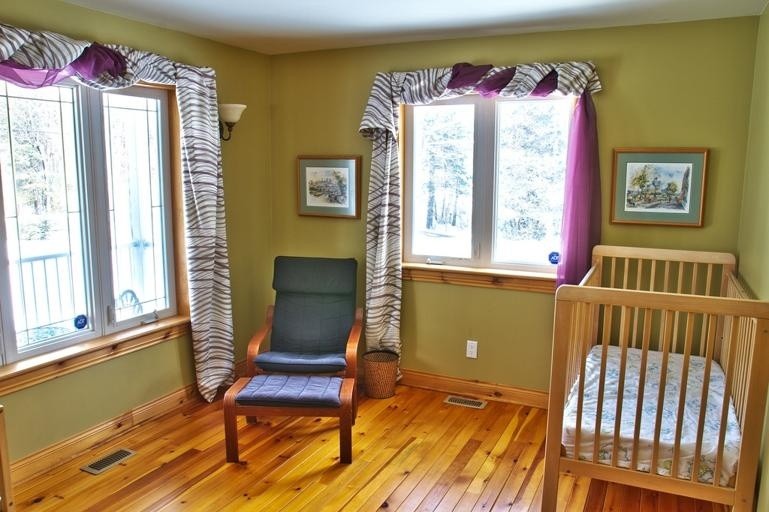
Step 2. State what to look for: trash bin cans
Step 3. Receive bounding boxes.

[361,349,400,399]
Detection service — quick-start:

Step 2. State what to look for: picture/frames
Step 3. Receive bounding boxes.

[296,154,362,220]
[610,147,709,227]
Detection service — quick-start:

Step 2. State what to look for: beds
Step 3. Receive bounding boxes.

[541,245,769,512]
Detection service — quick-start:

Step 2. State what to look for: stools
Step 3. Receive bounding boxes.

[224,376,356,463]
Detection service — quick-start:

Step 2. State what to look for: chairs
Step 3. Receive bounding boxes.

[249,255,363,416]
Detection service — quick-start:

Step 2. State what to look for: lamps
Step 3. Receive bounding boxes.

[218,103,247,141]
